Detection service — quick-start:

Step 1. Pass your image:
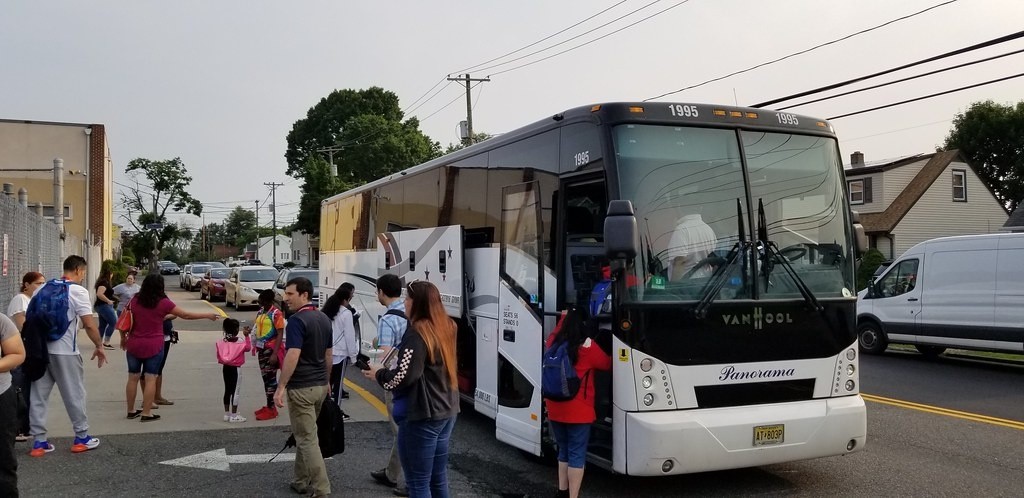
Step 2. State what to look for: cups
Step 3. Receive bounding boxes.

[369,349,384,367]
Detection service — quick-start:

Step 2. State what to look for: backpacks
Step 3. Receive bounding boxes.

[318,396,345,458]
[588,281,612,322]
[24,278,83,342]
[542,339,589,401]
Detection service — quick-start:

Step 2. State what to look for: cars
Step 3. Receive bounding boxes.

[158,262,227,291]
[225,265,281,310]
[271,268,319,317]
[200,267,234,301]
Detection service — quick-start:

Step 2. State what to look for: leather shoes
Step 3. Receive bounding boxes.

[392,486,409,497]
[371,468,397,488]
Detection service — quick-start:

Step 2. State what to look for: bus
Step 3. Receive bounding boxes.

[318,101,869,479]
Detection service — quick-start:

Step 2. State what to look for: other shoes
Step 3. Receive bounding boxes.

[14,433,34,441]
[141,414,161,421]
[127,409,141,419]
[310,493,329,498]
[141,401,158,409]
[341,389,350,399]
[289,482,307,493]
[604,415,613,425]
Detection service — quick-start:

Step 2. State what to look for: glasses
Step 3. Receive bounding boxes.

[408,279,418,294]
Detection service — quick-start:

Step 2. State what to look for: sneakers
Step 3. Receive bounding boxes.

[103,344,115,350]
[342,411,350,421]
[255,406,278,420]
[155,398,174,405]
[30,440,55,456]
[71,435,100,452]
[223,414,244,423]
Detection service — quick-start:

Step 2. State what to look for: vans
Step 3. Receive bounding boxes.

[854,231,1022,369]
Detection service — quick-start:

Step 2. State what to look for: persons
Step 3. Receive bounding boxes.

[120,274,222,423]
[361,281,462,498]
[322,281,360,423]
[666,195,718,283]
[215,318,252,423]
[273,277,332,498]
[540,309,612,498]
[361,274,416,497]
[243,289,285,420]
[0,314,27,498]
[25,255,109,456]
[94,260,120,350]
[5,272,46,440]
[113,273,141,318]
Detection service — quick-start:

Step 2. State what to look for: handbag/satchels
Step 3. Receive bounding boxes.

[115,297,133,331]
[170,330,179,344]
[14,384,29,433]
